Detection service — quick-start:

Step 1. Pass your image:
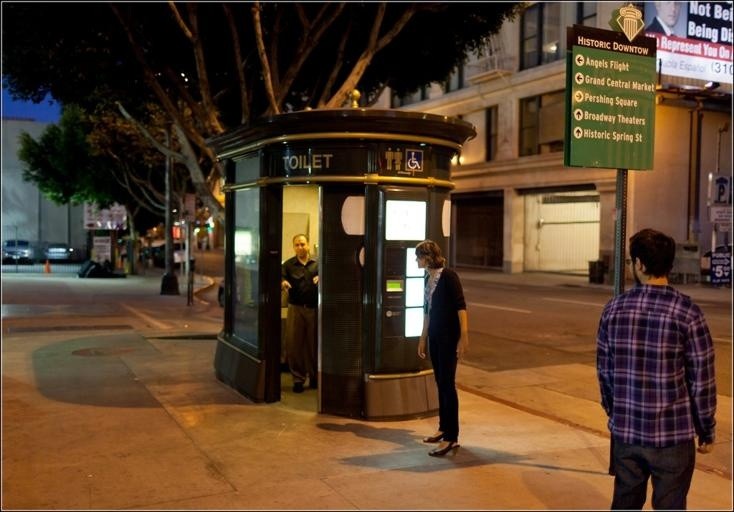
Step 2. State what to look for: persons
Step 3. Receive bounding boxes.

[280,233,319,394]
[415,240,468,457]
[596,227,716,512]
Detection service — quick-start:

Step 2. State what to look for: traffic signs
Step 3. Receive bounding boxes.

[566,20,654,171]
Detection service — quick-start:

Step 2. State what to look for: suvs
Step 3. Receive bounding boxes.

[2,240,35,266]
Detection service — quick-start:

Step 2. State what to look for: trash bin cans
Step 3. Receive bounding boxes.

[588,261,605,283]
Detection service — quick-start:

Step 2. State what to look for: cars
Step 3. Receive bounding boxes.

[456,224,502,267]
[40,243,75,262]
[117,238,196,269]
[216,277,225,306]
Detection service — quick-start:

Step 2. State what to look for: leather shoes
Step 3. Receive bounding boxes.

[292,381,305,393]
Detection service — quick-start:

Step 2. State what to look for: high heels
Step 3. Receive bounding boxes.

[427,441,461,458]
[422,432,446,444]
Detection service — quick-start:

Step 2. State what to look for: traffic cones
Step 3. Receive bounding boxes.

[41,259,52,275]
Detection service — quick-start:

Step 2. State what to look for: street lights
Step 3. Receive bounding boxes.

[153,114,185,295]
